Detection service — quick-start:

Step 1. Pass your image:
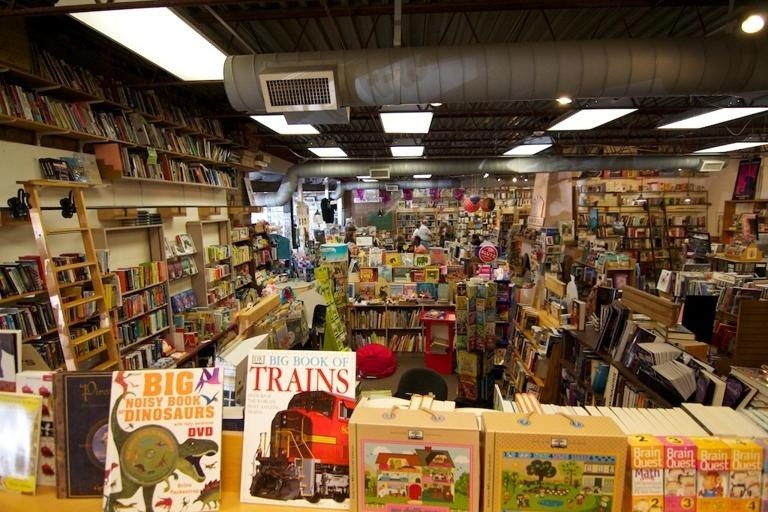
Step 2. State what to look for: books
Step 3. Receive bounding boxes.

[235,299,307,349]
[230,225,289,302]
[657,262,768,299]
[579,207,648,225]
[110,260,169,369]
[430,337,449,354]
[0,47,118,141]
[726,364,768,409]
[352,331,427,355]
[351,253,449,305]
[238,350,358,512]
[119,78,271,190]
[0,249,110,300]
[487,187,533,235]
[454,215,489,247]
[394,212,416,241]
[573,300,726,408]
[39,156,84,182]
[572,253,634,290]
[454,281,508,406]
[1,300,110,372]
[575,168,707,190]
[203,242,230,301]
[669,227,709,262]
[505,304,547,402]
[163,232,200,312]
[578,192,707,206]
[174,311,225,349]
[122,210,163,226]
[576,227,651,250]
[82,152,103,186]
[348,307,423,329]
[0,366,223,512]
[667,212,706,226]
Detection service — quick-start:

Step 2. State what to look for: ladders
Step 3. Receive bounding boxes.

[23,178,125,374]
[645,199,673,286]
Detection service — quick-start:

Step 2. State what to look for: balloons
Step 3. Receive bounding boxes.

[464,195,494,213]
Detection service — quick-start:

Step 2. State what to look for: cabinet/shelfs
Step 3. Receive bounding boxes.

[0,41,237,190]
[483,189,533,209]
[507,275,571,404]
[574,172,708,284]
[721,202,764,273]
[570,240,635,294]
[393,205,461,246]
[463,205,520,250]
[0,221,312,370]
[452,277,512,404]
[530,227,562,275]
[0,303,759,512]
[345,250,512,358]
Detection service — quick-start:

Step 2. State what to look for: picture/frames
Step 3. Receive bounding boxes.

[731,160,759,202]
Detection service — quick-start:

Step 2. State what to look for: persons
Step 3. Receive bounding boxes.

[742,218,755,240]
[411,219,449,252]
[343,227,359,255]
[308,212,327,238]
